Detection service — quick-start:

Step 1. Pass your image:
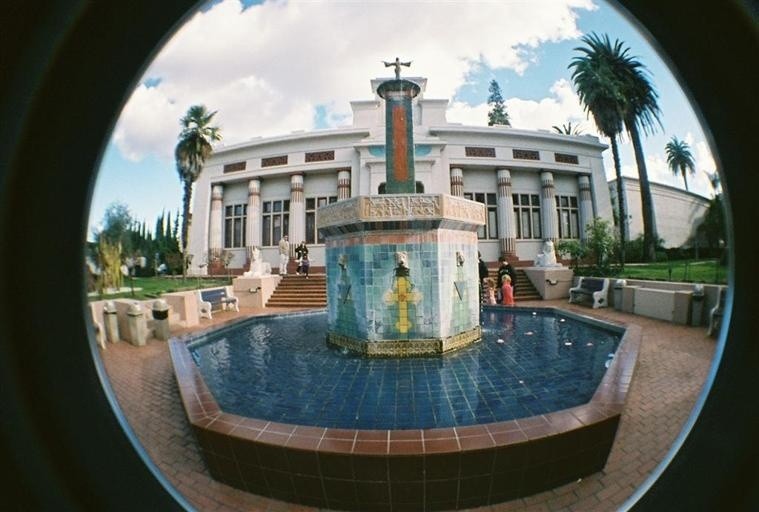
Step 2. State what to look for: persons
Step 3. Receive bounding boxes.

[302,255,309,279]
[478,251,516,306]
[295,241,308,274]
[381,58,414,80]
[278,235,289,275]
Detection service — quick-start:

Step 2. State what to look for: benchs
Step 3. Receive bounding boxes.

[566,272,611,311]
[196,286,240,322]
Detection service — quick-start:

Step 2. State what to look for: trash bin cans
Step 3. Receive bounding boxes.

[127,302,147,347]
[613,278,626,310]
[150,299,170,340]
[102,301,119,345]
[688,283,705,327]
